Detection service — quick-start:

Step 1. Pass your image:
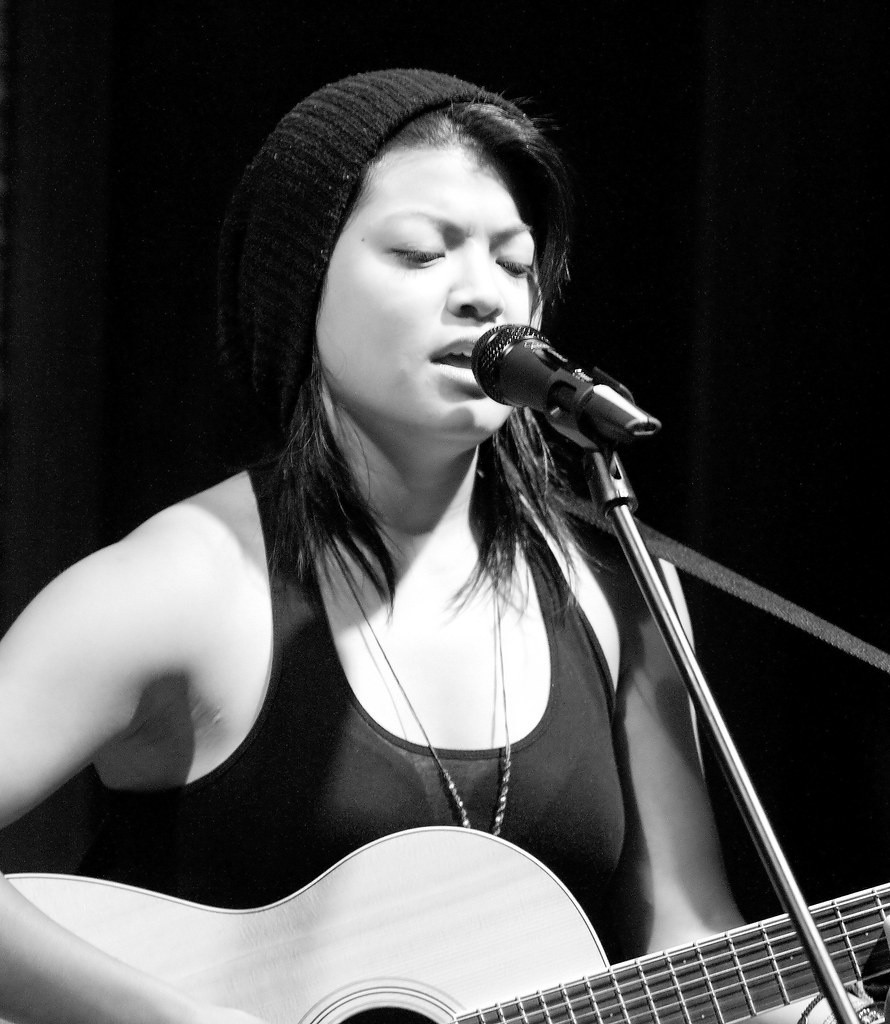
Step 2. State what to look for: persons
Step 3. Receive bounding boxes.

[0,71,890,1024]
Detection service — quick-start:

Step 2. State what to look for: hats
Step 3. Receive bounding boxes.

[217,70,527,429]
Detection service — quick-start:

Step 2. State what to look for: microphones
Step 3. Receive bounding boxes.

[471,325,661,437]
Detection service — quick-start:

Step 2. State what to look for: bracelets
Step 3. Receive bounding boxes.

[796,984,886,1024]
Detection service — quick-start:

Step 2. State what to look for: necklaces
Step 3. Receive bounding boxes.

[324,532,511,837]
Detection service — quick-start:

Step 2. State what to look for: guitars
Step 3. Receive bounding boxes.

[0,820,890,1024]
